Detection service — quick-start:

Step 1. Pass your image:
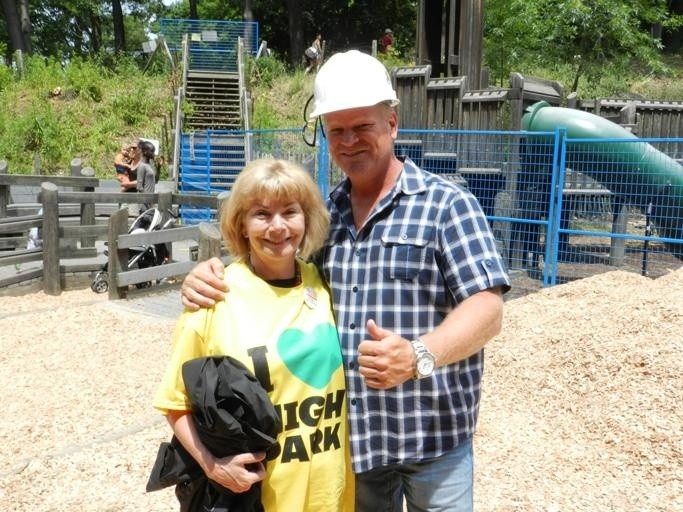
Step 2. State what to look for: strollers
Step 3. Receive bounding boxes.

[92,207,179,293]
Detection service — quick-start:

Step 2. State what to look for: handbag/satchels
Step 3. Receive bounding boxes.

[305,48,318,59]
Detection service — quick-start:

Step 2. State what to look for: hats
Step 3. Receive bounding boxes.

[385,29,392,33]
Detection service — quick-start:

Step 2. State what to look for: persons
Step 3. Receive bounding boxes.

[114,139,169,284]
[180,49,511,512]
[154,158,357,512]
[380,28,395,56]
[304,34,322,74]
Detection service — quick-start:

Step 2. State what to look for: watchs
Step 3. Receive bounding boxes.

[410,339,435,382]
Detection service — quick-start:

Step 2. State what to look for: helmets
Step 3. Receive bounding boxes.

[309,50,400,119]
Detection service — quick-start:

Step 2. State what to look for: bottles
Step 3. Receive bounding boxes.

[162,257,168,263]
